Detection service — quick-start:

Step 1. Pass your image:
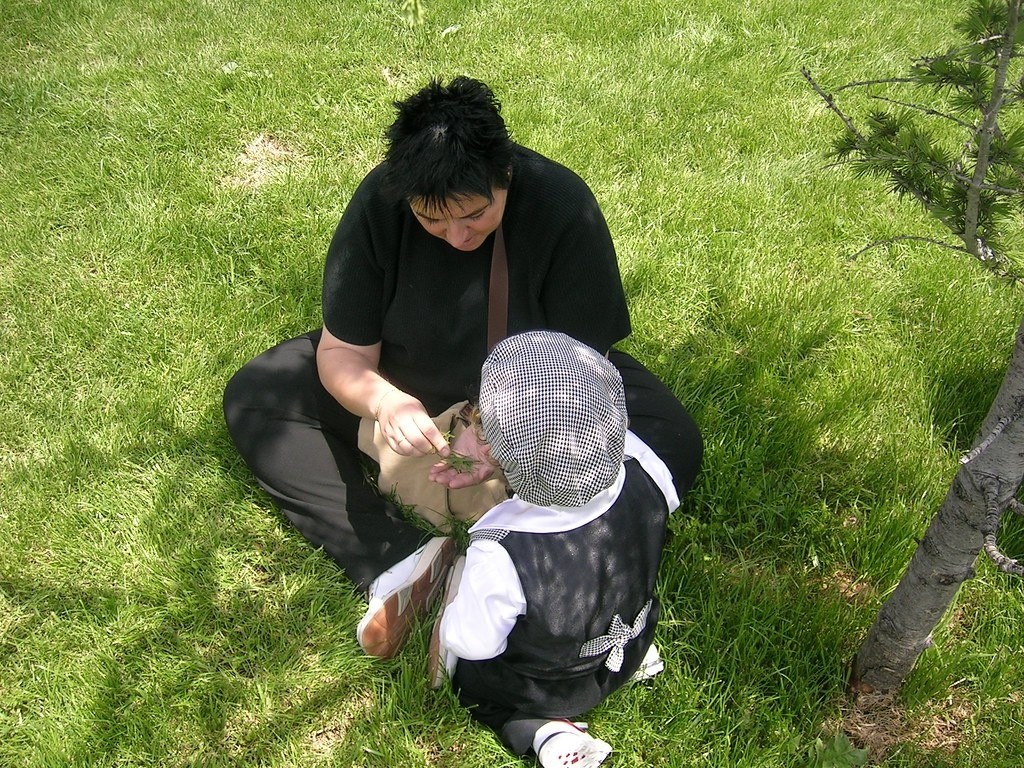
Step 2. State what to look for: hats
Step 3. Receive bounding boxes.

[480,331,627,506]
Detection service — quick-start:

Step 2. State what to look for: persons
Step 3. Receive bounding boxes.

[438,331,686,768]
[221,76,704,655]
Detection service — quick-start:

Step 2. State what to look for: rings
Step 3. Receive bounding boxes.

[398,437,406,445]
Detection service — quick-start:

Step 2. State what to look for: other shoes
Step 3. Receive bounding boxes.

[622,642,664,689]
[533,718,611,768]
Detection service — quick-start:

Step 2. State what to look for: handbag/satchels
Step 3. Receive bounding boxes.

[357,400,510,535]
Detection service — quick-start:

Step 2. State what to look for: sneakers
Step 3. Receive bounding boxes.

[356,535,460,664]
[424,555,466,691]
